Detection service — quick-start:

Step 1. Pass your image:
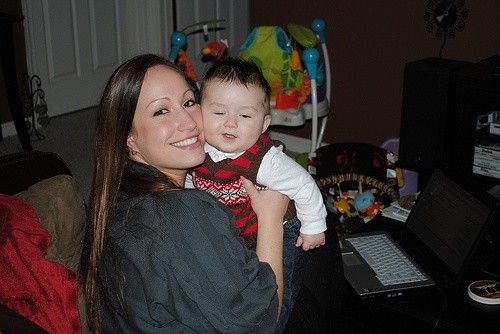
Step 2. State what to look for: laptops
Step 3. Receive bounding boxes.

[338,168,494,299]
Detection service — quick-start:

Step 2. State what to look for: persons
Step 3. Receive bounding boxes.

[184,57,328,334]
[76,54,290,334]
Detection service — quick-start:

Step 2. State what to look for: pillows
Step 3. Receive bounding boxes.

[13,175,93,334]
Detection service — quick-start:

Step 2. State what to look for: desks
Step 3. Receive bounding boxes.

[283,142,500,334]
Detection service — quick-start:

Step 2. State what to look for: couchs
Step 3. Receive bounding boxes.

[0,149,99,334]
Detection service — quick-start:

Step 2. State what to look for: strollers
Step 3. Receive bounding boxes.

[168,17,331,166]
